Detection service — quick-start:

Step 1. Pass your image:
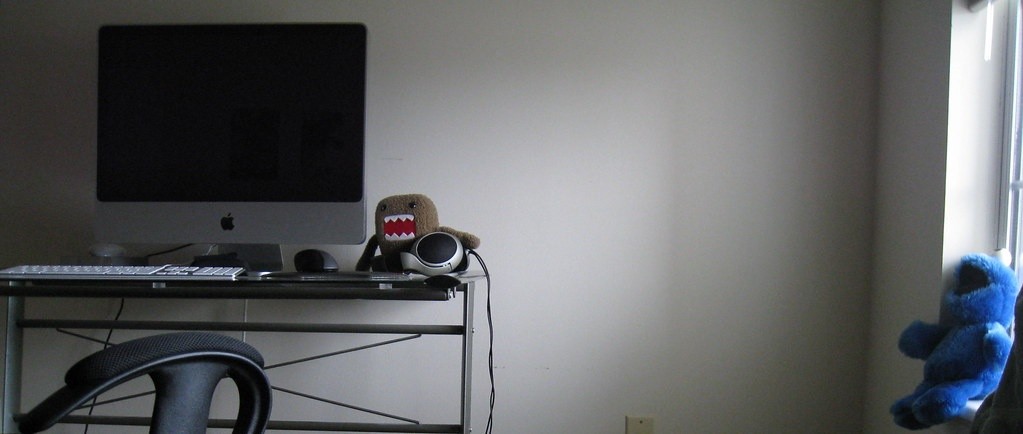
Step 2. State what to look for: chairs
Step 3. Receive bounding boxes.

[18,332,273,434]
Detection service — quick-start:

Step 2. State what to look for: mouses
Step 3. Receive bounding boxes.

[293,249,339,274]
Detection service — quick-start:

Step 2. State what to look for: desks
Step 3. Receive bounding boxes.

[0,275,493,434]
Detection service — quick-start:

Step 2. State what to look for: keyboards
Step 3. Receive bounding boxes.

[0,266,246,282]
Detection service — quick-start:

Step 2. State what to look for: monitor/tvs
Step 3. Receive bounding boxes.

[94,21,368,276]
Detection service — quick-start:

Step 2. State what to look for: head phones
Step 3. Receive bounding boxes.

[401,230,470,277]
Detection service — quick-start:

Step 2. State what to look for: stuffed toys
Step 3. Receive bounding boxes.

[888,253,1023,429]
[356,194,480,272]
